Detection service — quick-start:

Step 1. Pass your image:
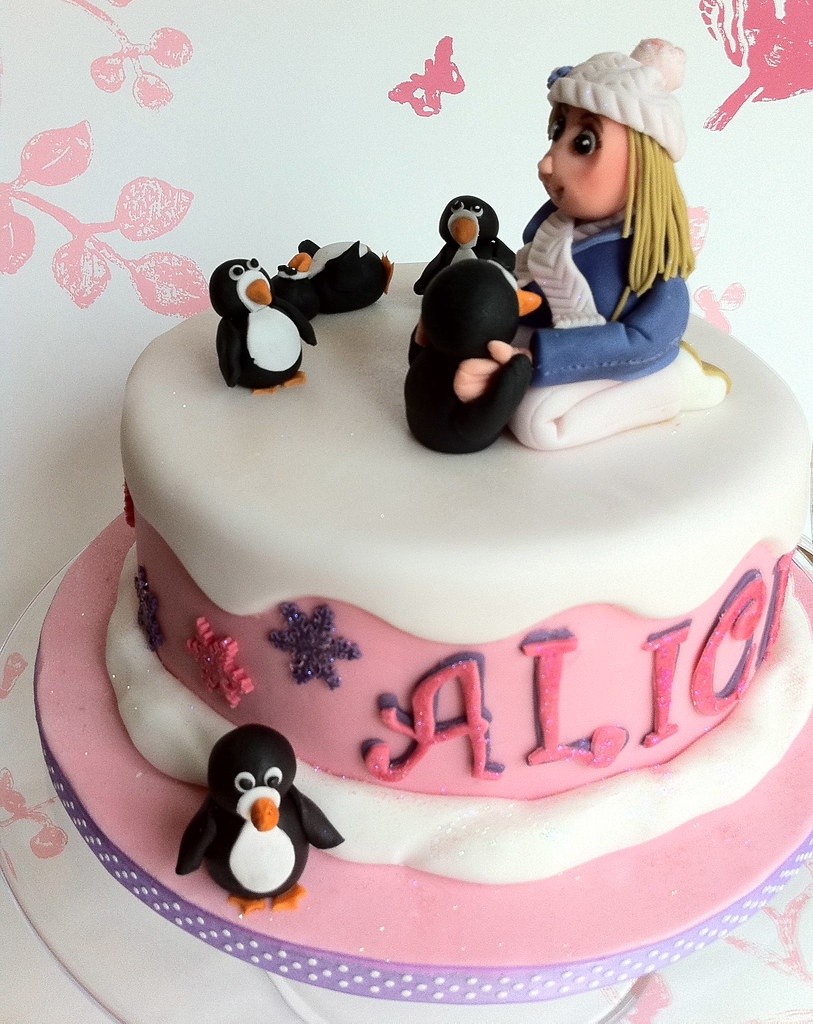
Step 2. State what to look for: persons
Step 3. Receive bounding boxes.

[507,36,726,449]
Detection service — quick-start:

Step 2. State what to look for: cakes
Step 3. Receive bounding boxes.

[105,40,812,917]
